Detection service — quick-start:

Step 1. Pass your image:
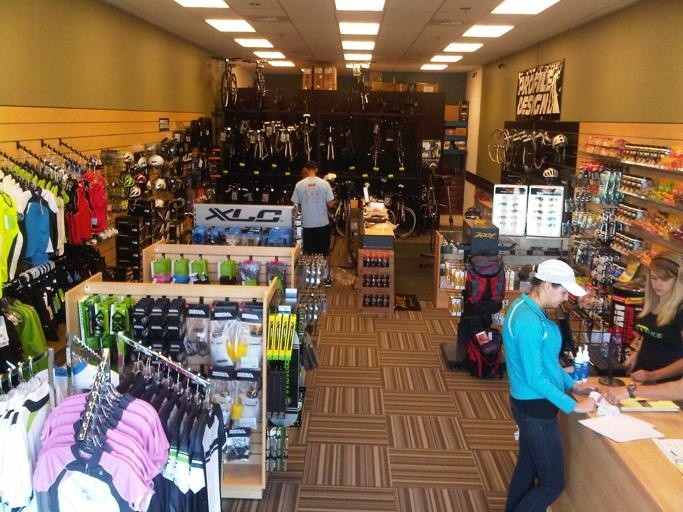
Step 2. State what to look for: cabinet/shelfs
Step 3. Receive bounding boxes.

[570,141,683,325]
[342,199,567,331]
[62,200,308,502]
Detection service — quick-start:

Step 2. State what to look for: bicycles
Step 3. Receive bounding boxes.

[487,120,552,174]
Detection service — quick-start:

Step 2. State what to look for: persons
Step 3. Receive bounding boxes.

[503,259,600,512]
[622,250,683,383]
[290,162,335,287]
[607,378,683,405]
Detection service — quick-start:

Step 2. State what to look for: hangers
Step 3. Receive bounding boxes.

[0,142,104,207]
[1,256,63,312]
[0,356,51,431]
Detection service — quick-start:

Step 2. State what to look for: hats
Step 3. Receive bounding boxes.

[534,259,587,296]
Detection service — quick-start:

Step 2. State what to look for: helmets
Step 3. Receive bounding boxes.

[148,155,164,166]
[154,178,166,190]
[543,168,558,177]
[121,151,147,198]
[155,199,164,208]
[553,134,567,146]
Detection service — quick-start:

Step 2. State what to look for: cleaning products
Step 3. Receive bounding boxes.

[572,344,591,384]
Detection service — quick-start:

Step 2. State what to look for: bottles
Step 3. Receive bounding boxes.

[362,256,389,307]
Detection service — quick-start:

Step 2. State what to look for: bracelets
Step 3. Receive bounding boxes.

[634,349,641,353]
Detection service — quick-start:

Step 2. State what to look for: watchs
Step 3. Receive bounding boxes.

[627,384,636,397]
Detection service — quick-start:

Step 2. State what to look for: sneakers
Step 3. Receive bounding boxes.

[324,280,331,287]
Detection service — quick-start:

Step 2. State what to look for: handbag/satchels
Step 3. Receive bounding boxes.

[469,328,502,377]
[464,256,505,314]
